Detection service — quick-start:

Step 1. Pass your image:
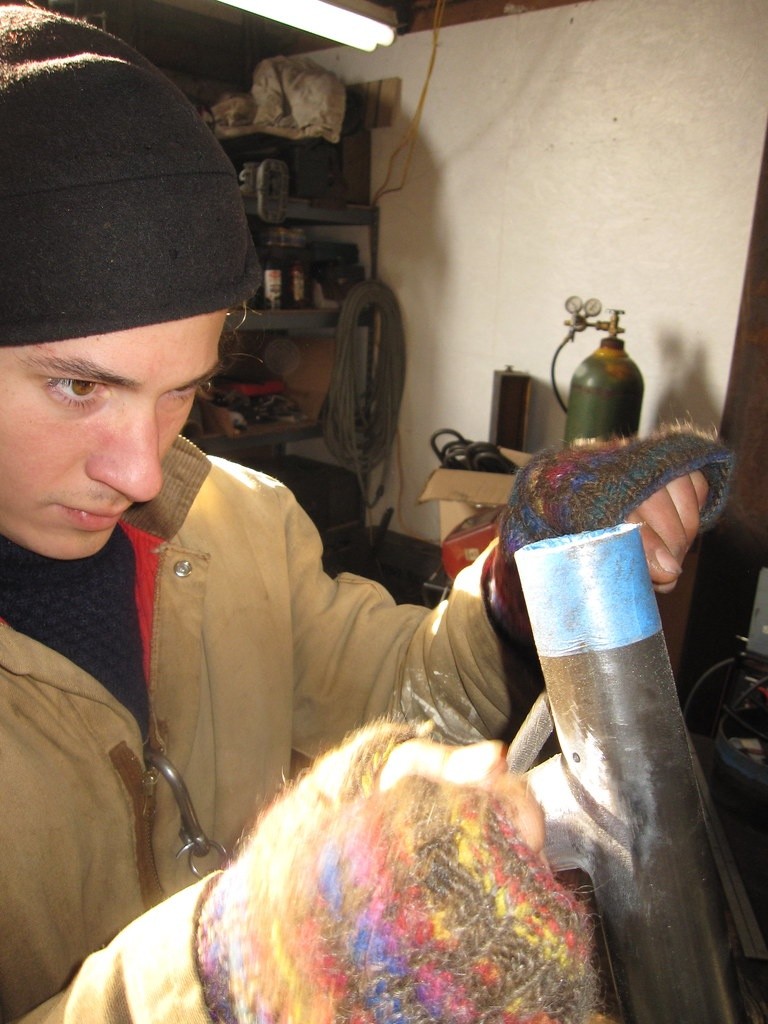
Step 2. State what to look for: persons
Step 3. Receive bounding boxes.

[0,0,732,1024]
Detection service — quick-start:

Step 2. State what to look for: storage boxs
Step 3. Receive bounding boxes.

[416,448,534,548]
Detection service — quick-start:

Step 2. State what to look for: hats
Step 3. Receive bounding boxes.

[0,6,262,349]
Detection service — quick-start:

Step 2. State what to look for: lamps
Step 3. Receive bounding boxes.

[219,0,399,52]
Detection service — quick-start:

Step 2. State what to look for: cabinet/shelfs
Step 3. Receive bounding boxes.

[193,194,380,566]
